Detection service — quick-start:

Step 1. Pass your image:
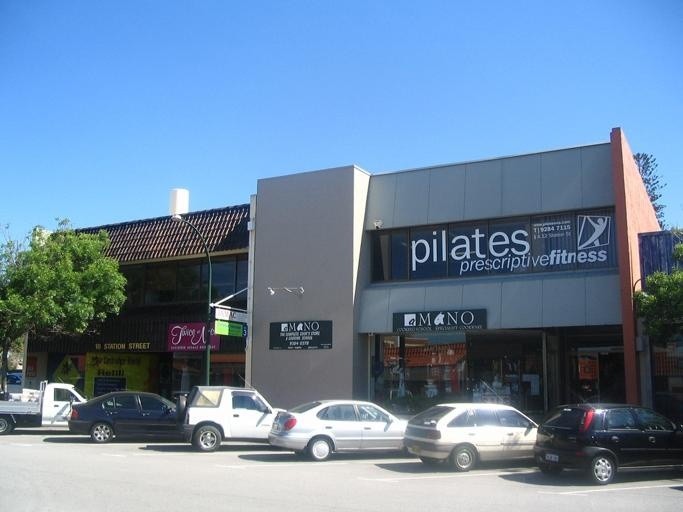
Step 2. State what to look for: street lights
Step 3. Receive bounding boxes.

[168,188,212,388]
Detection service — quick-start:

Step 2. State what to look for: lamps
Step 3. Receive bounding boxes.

[266,286,305,297]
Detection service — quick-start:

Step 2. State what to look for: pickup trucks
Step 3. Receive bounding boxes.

[0,379,88,435]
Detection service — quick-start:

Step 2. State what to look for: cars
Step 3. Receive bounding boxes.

[404,401,538,471]
[67,391,185,443]
[178,382,281,451]
[266,399,407,461]
[535,403,682,486]
